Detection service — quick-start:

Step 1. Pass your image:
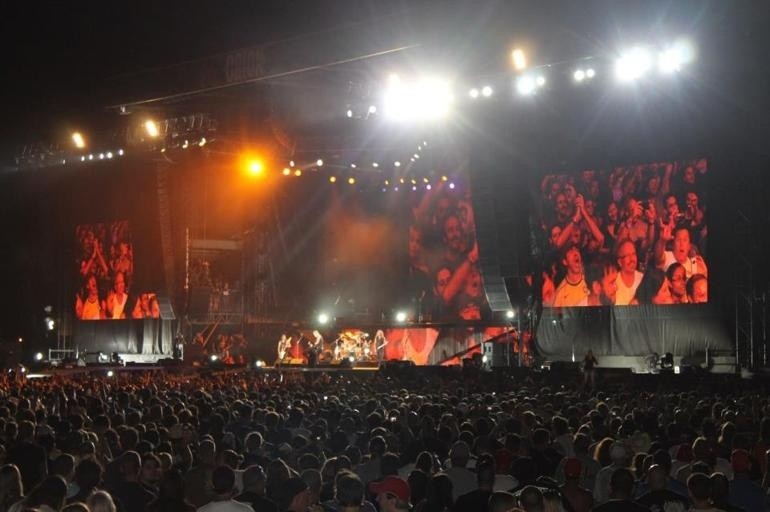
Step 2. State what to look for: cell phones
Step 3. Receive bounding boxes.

[691,257,698,274]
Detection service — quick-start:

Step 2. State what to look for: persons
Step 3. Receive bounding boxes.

[75,222,163,319]
[308,328,326,367]
[276,332,291,365]
[297,328,314,360]
[410,189,485,320]
[0,373,769,511]
[580,350,598,387]
[173,331,187,357]
[528,155,711,305]
[335,327,391,367]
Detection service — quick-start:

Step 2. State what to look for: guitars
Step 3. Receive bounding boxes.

[279,337,291,359]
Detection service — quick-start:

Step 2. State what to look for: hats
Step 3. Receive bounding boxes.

[560,456,582,471]
[368,476,410,500]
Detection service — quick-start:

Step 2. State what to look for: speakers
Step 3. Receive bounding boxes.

[143,170,179,321]
[379,360,414,370]
[188,286,211,317]
[485,342,505,366]
[469,156,517,313]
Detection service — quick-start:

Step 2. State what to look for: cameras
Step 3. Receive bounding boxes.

[640,200,649,210]
